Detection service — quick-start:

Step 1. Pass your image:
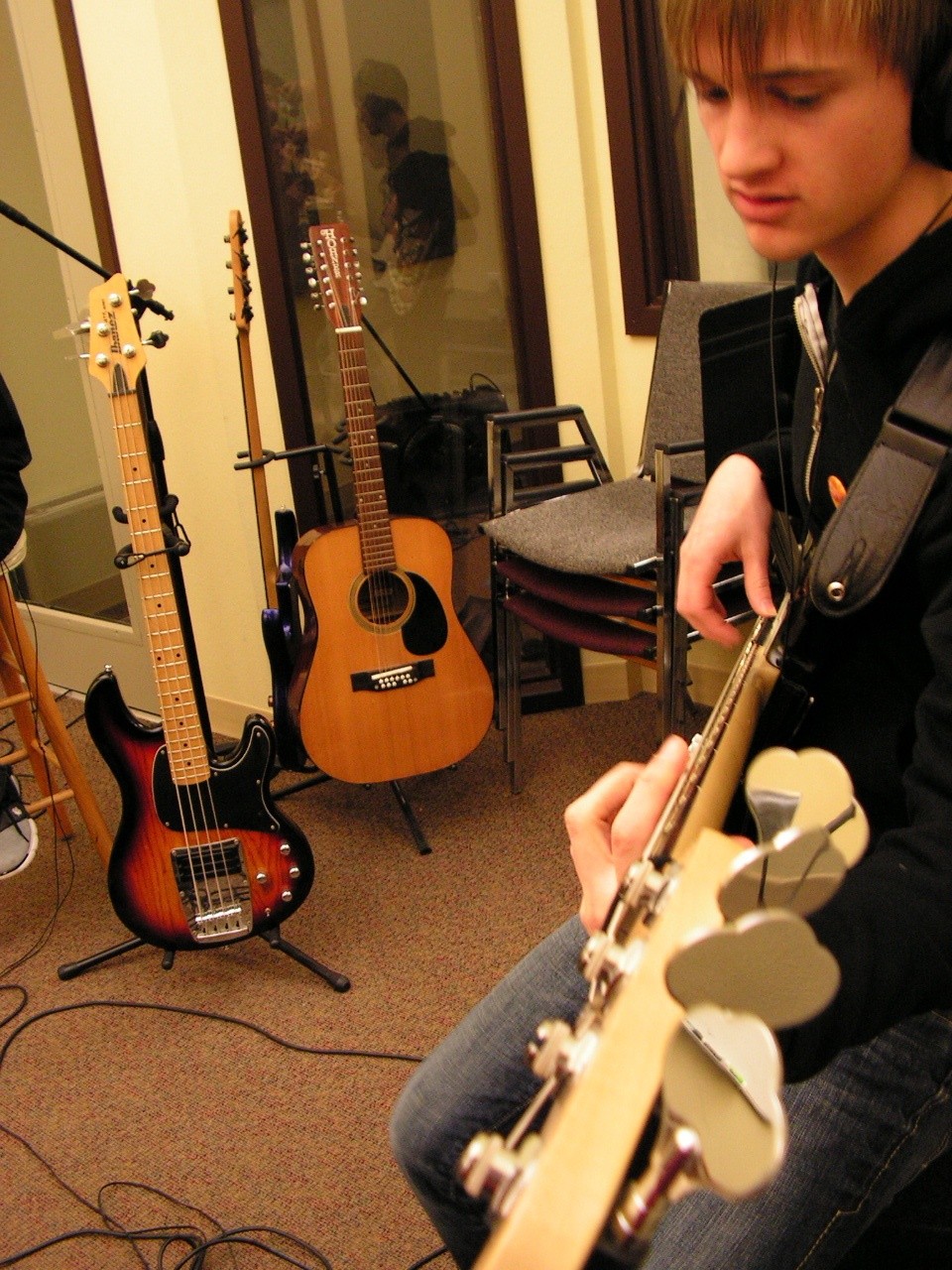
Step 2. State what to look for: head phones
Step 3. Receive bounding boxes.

[909,18,952,172]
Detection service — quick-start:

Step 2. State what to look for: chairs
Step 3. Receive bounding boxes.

[476,278,804,797]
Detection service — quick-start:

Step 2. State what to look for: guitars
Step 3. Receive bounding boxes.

[221,208,307,772]
[85,271,319,947]
[288,220,499,788]
[452,580,880,1270]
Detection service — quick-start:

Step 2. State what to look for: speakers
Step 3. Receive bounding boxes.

[368,391,512,519]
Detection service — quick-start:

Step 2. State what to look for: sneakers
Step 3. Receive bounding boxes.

[0,765,38,879]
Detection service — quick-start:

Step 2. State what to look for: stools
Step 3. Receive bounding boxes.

[0,528,115,867]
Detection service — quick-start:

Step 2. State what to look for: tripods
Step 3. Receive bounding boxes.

[0,198,459,993]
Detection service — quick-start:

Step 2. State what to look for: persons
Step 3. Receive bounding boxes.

[0,375,39,878]
[387,0,952,1270]
